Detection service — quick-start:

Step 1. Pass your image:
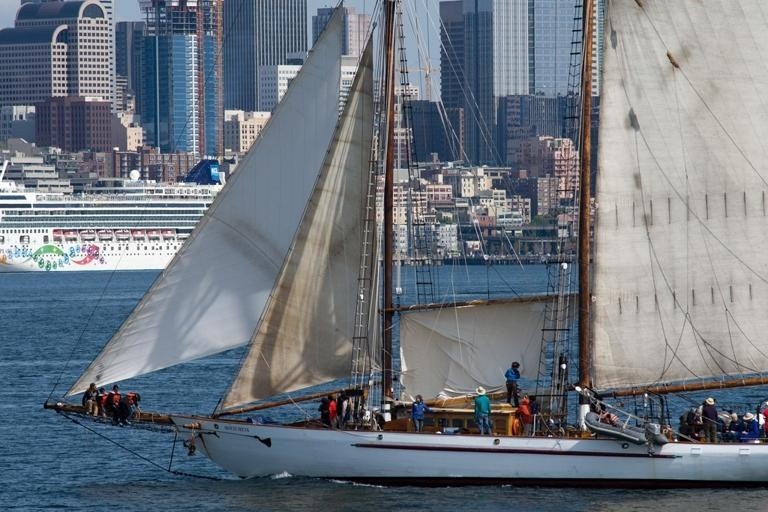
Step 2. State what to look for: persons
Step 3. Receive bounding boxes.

[686,397,768,444]
[474,386,492,435]
[317,388,385,433]
[410,395,432,432]
[516,395,538,437]
[81,382,141,427]
[505,362,520,407]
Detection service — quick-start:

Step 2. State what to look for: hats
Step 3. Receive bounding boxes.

[113,385,118,390]
[137,393,140,401]
[706,397,715,405]
[99,388,105,392]
[732,414,737,421]
[743,413,754,420]
[90,383,95,387]
[476,386,486,395]
[321,394,336,404]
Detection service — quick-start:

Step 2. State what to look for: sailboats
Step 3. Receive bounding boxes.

[45,0,767,487]
